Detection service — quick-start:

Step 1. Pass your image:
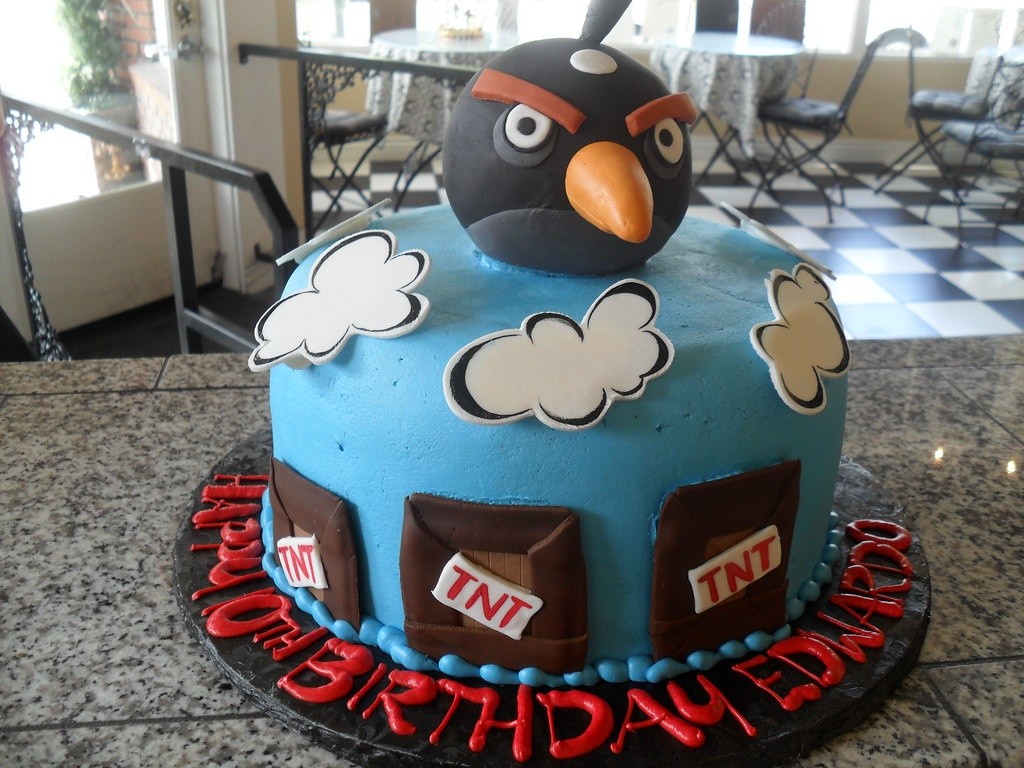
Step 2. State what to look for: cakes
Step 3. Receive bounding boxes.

[258,0,853,686]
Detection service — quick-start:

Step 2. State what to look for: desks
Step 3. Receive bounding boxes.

[646,34,806,195]
[360,24,545,213]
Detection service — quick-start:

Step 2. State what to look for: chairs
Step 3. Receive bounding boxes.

[295,0,1024,252]
